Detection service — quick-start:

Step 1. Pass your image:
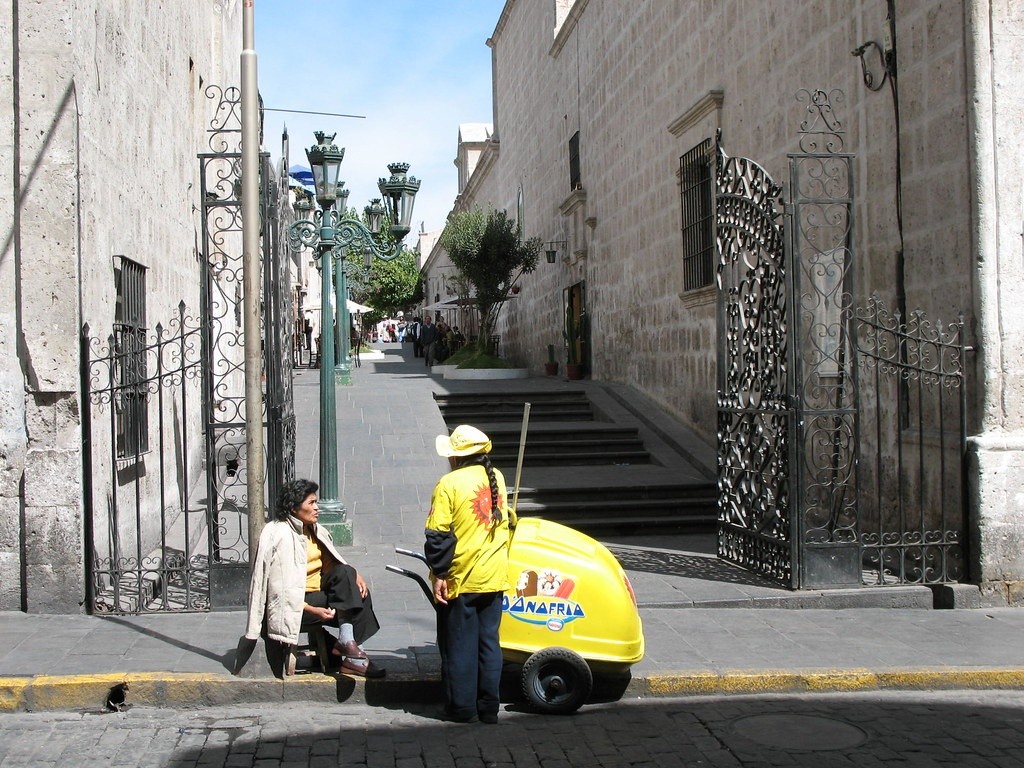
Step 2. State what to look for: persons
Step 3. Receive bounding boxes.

[424,425,510,725]
[316,315,461,367]
[245,479,386,678]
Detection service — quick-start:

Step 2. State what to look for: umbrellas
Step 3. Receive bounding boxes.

[289,164,315,187]
[421,289,518,351]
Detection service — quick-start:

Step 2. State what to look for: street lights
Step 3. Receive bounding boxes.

[287,130,422,547]
[333,178,385,377]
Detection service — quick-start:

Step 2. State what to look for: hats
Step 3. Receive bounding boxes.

[436,425,492,457]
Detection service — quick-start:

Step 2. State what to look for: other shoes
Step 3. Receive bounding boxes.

[478,710,498,723]
[436,708,479,722]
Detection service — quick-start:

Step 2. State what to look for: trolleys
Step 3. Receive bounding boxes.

[386,516,646,717]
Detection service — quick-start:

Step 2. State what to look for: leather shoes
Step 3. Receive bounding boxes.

[332,640,367,659]
[340,658,386,679]
